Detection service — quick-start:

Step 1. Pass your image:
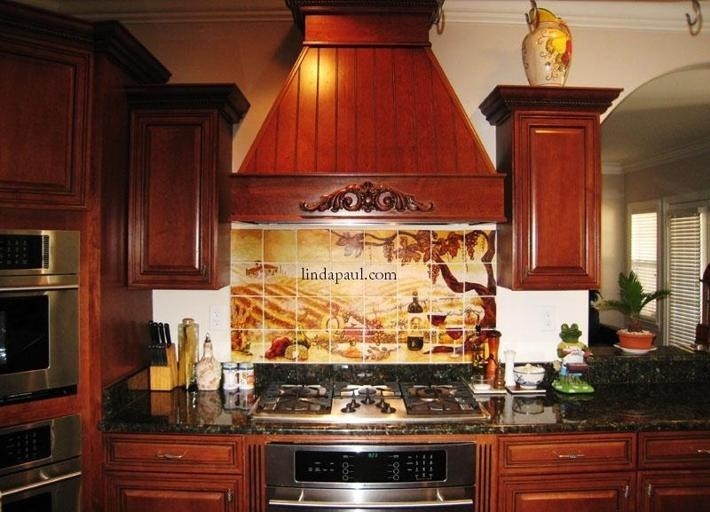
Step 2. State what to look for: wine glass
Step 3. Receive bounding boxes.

[427,314,449,347]
[444,326,466,358]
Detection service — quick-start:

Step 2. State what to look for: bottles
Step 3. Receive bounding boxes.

[470,324,516,390]
[196,392,222,426]
[194,334,221,391]
[174,389,196,433]
[407,292,424,351]
[176,317,200,392]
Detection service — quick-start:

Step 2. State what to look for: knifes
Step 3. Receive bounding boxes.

[147,320,171,367]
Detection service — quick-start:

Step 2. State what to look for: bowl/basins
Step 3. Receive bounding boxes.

[512,399,546,415]
[511,366,546,389]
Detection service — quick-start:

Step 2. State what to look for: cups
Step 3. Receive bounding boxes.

[232,390,255,411]
[222,391,239,414]
[232,361,255,389]
[223,363,238,390]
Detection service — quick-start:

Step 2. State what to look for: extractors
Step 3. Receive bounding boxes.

[222,0,511,230]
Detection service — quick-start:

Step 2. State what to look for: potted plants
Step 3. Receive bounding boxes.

[592,271,672,351]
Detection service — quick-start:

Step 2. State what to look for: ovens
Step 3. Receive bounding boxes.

[0,413,83,512]
[263,439,476,512]
[0,227,78,403]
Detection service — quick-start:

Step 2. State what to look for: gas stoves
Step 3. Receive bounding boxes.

[246,376,491,426]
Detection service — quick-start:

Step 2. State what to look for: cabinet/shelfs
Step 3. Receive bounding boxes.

[102,434,267,512]
[127,83,251,291]
[497,431,710,511]
[479,85,624,292]
